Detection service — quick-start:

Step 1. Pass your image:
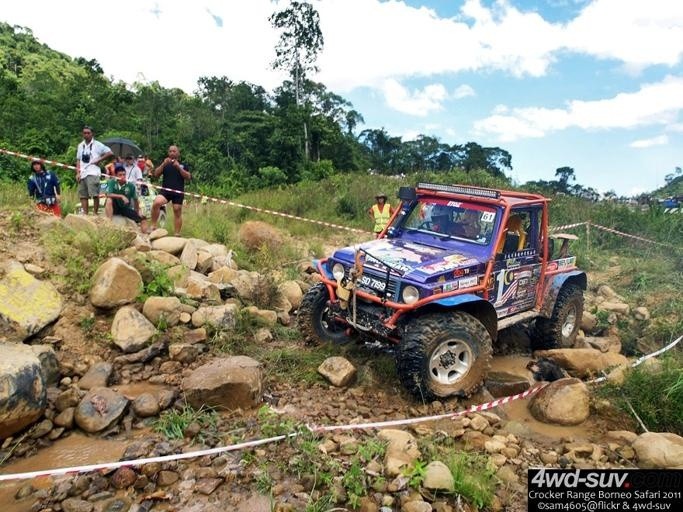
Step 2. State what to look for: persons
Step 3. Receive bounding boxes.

[368,192,396,239]
[74,127,113,217]
[98,150,153,236]
[27,160,62,217]
[150,145,192,238]
[418,199,437,229]
[425,204,467,238]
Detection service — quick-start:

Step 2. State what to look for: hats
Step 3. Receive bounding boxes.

[375,193,387,201]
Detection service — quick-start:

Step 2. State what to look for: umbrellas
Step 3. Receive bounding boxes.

[101,138,143,158]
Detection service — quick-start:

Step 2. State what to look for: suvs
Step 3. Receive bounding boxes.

[297,181,587,402]
[73,179,167,229]
